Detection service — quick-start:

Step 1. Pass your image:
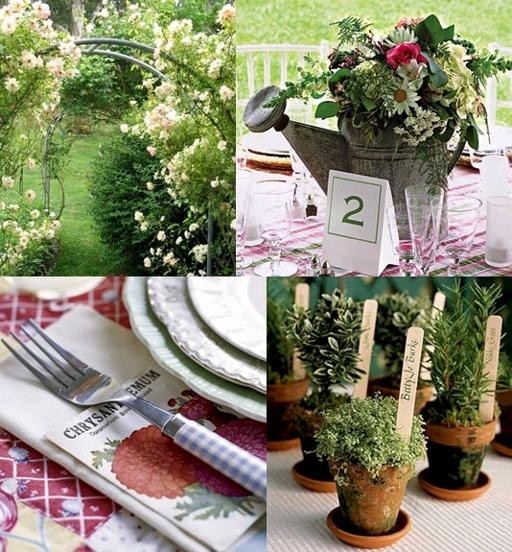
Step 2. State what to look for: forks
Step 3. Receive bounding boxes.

[0,318,268,500]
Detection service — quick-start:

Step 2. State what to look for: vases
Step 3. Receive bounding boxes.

[339,144,466,245]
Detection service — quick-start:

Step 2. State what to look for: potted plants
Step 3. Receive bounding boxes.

[267,276,512,550]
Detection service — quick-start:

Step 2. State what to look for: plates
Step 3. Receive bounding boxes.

[121,276,266,426]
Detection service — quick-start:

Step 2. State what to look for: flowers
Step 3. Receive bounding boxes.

[262,13,512,196]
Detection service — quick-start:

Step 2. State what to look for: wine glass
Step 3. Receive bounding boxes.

[384,182,482,277]
[235,168,300,278]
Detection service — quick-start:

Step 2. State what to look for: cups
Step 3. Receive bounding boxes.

[485,198,512,267]
